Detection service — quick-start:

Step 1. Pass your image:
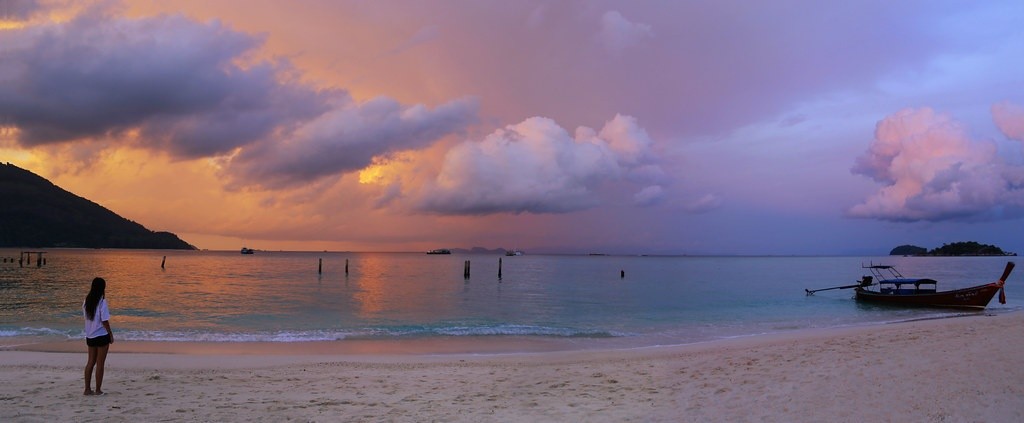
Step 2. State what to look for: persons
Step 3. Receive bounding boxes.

[83,277,114,395]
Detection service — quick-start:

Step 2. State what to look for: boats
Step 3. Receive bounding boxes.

[506,248,523,256]
[427,248,451,255]
[852,260,1017,311]
[241,248,254,254]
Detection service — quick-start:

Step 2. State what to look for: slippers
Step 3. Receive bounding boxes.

[94,392,104,396]
